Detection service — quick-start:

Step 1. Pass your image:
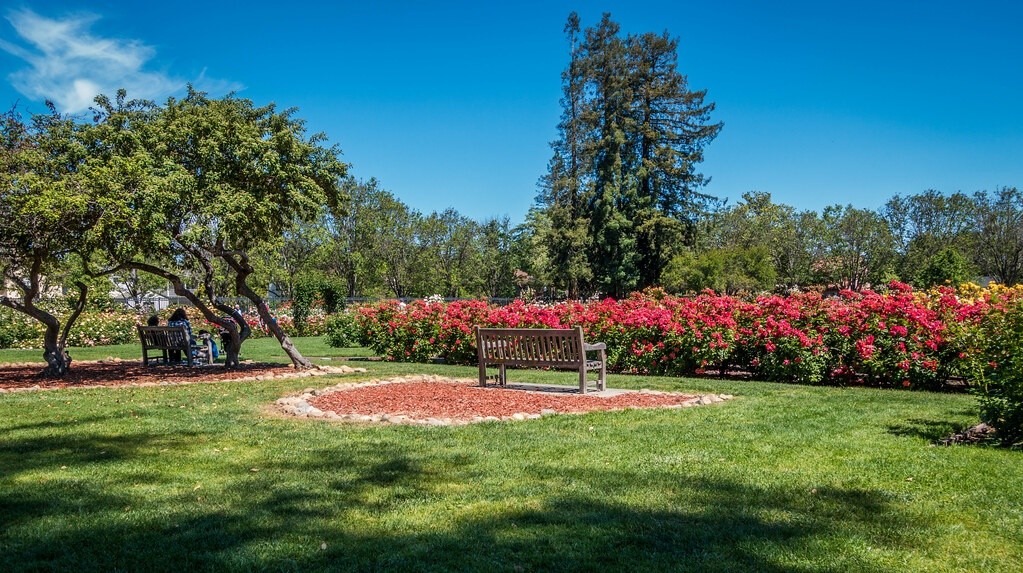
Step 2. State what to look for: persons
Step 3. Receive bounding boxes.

[234,305,241,315]
[259,312,277,335]
[198,330,219,361]
[147,315,160,326]
[168,308,202,366]
[399,299,407,315]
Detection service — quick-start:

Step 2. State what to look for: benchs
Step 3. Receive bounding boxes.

[473,324,607,395]
[135,324,209,369]
